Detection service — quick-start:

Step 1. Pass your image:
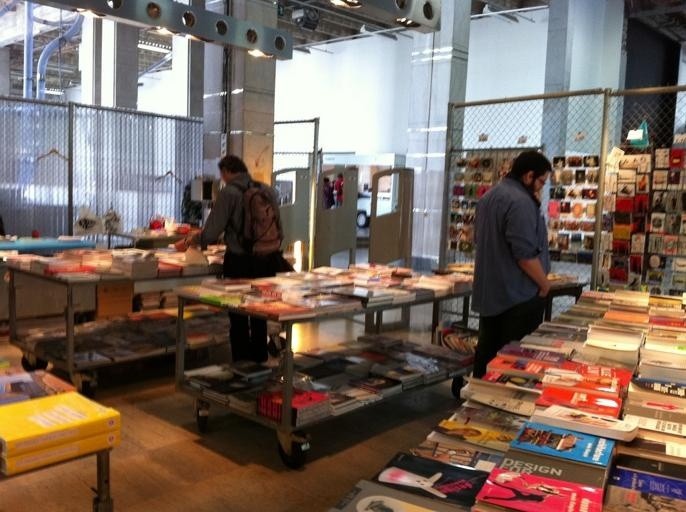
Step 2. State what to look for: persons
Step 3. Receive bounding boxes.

[168,154,284,361]
[435,425,481,438]
[324,177,335,208]
[474,151,553,381]
[335,174,344,207]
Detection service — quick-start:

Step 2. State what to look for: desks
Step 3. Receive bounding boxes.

[431,262,588,342]
[108,227,201,249]
[0,237,96,256]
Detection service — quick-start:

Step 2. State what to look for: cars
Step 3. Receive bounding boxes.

[356,191,390,228]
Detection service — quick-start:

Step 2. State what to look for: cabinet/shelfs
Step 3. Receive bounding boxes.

[0,341,120,512]
[174,265,480,470]
[331,290,686,512]
[4,250,296,398]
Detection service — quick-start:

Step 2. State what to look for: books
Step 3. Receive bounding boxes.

[18,311,166,365]
[0,369,121,474]
[183,331,472,416]
[338,289,686,511]
[175,263,474,321]
[9,247,224,280]
[598,148,686,291]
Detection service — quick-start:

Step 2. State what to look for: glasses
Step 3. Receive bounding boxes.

[536,175,546,184]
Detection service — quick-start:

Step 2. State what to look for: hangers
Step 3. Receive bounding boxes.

[37,149,68,162]
[155,171,182,183]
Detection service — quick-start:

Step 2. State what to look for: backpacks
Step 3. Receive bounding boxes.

[231,182,281,255]
